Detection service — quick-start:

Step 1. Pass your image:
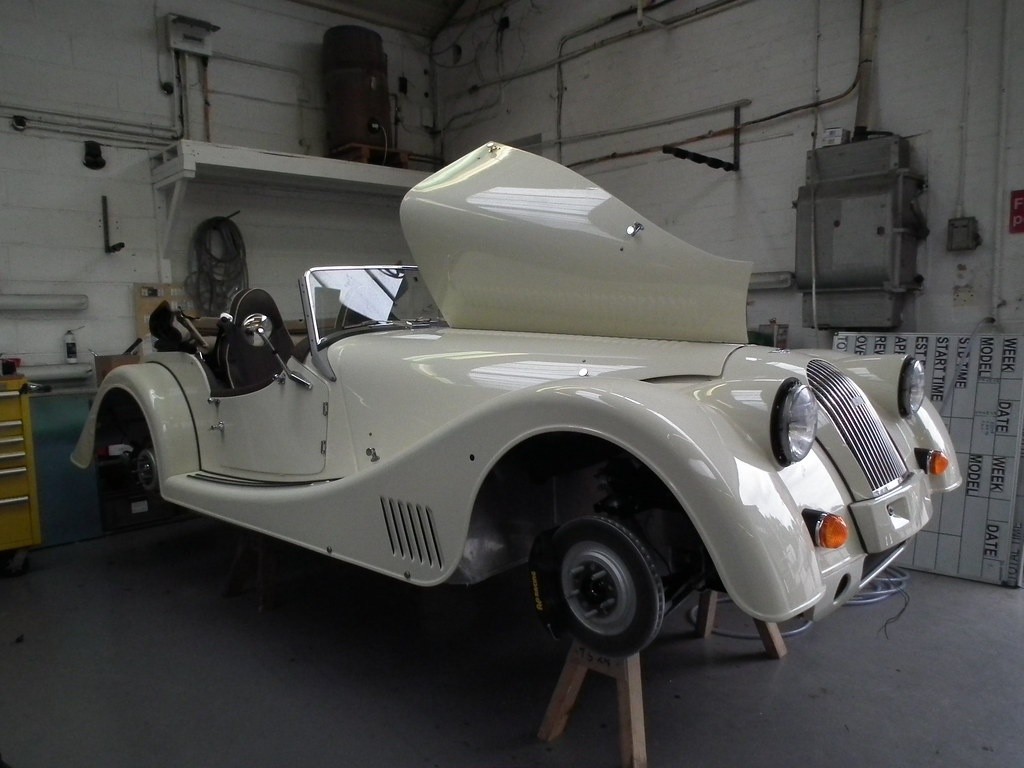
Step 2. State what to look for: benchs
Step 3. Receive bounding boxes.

[531,586,787,768]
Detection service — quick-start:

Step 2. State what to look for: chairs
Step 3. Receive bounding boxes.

[332,283,400,333]
[214,289,297,393]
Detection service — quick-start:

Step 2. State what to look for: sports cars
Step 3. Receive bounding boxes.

[68,140,965,661]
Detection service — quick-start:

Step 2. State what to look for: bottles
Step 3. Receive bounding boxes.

[64,329,78,364]
